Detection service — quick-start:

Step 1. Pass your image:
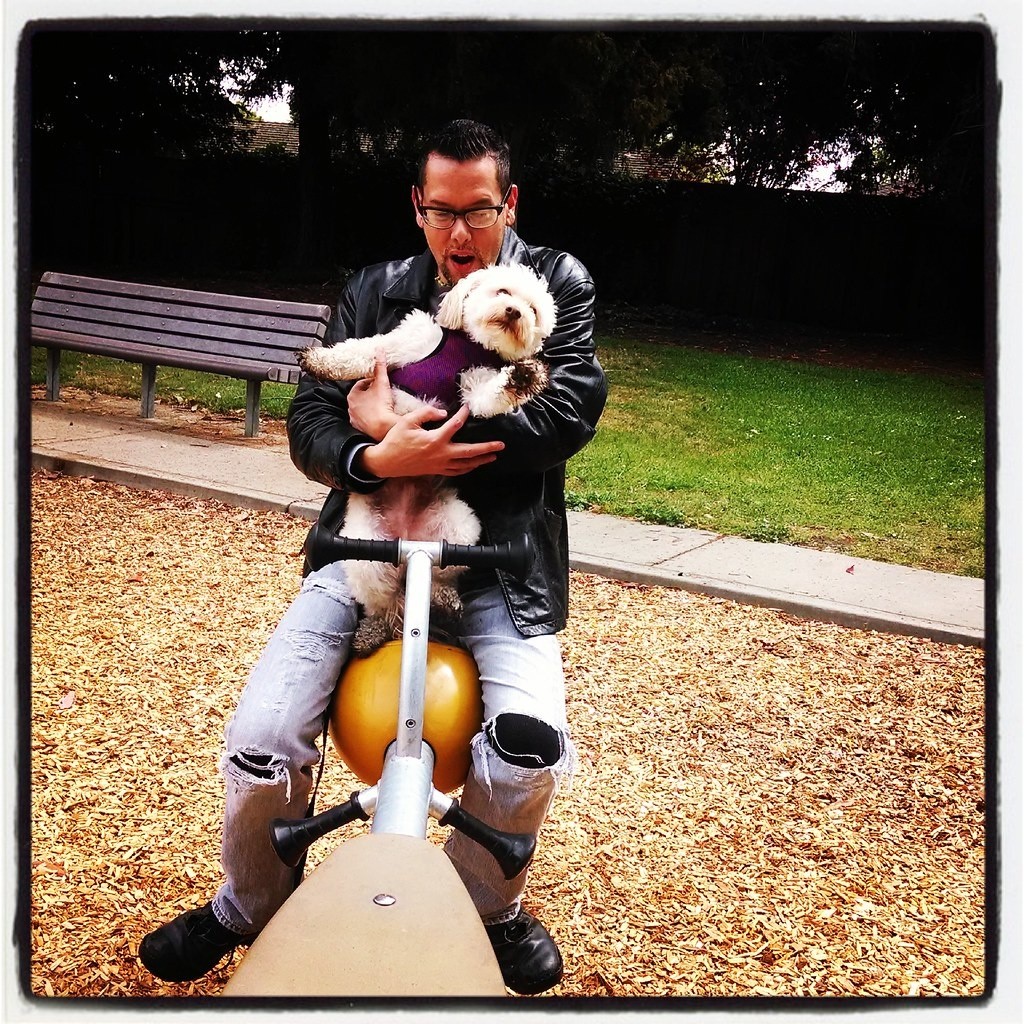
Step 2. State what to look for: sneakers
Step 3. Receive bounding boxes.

[486,910,564,995]
[139,902,262,983]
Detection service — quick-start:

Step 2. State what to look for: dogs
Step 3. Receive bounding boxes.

[293,264,558,659]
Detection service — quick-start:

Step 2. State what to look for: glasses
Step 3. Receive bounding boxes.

[414,184,512,230]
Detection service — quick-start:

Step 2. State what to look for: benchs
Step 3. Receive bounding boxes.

[33,269,336,436]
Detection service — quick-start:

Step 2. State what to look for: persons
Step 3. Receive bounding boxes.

[140,118,607,994]
